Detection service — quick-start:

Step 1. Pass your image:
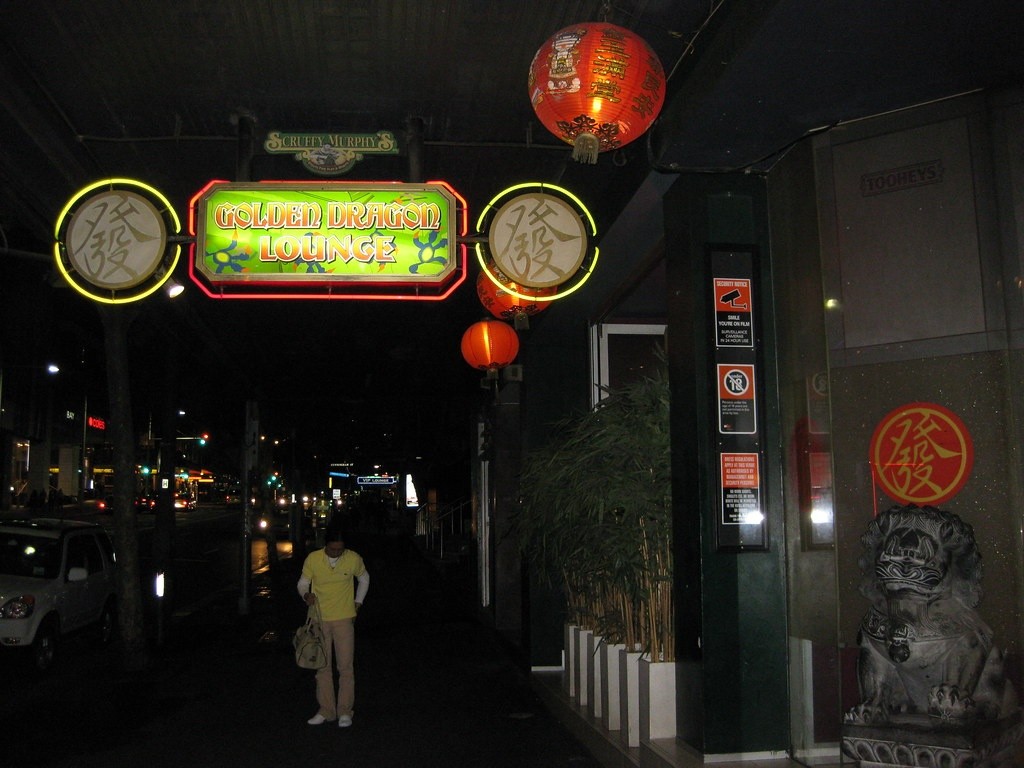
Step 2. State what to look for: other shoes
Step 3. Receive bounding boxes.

[338,714,353,727]
[307,713,325,725]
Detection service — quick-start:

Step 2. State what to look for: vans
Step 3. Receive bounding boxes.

[226,483,243,509]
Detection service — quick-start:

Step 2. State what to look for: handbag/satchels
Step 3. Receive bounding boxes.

[293,597,328,669]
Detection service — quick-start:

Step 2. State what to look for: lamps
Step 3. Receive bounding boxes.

[476,256,558,331]
[461,310,520,381]
[528,11,668,164]
[152,265,185,299]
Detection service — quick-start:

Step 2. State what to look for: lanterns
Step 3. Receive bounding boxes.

[460,316,519,379]
[529,22,667,166]
[477,257,558,330]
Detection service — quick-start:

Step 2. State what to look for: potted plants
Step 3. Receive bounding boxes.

[501,344,675,749]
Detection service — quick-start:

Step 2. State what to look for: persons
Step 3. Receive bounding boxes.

[304,534,370,729]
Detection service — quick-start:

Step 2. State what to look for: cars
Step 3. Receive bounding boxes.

[135,494,158,514]
[102,494,113,515]
[0,519,117,670]
[175,493,196,512]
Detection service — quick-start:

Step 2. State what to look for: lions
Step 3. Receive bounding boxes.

[841,504,1017,733]
